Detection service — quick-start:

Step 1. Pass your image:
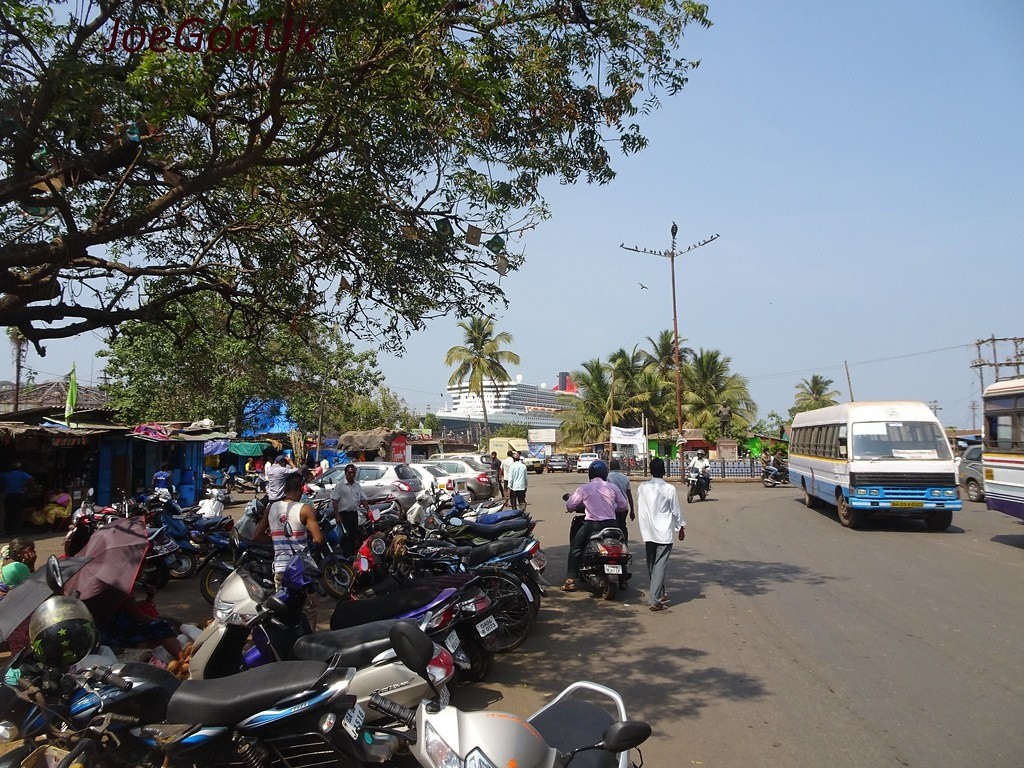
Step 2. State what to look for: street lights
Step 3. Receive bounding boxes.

[620,220,721,484]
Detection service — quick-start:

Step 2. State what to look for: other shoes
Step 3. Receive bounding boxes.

[651,604,667,611]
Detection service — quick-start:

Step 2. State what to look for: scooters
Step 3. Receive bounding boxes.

[685,465,713,503]
[356,620,654,768]
[561,493,633,601]
[759,461,790,488]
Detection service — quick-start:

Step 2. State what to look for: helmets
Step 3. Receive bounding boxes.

[696,449,705,457]
[28,596,96,668]
[589,460,609,481]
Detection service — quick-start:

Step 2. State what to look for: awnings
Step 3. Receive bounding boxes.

[128,428,242,442]
[203,439,273,457]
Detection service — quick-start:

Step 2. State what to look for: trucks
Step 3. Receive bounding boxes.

[489,437,544,474]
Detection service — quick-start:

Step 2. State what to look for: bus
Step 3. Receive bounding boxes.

[981,377,1024,524]
[787,400,963,532]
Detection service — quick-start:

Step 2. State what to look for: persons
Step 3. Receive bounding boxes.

[560,460,629,591]
[773,448,789,484]
[0,462,34,538]
[0,536,37,602]
[217,455,330,494]
[637,457,686,612]
[67,524,181,658]
[332,457,341,468]
[717,401,732,439]
[491,451,505,500]
[686,449,711,491]
[761,448,779,481]
[607,460,635,549]
[252,472,324,595]
[151,462,173,489]
[568,448,609,465]
[500,449,528,513]
[676,449,681,460]
[330,464,372,558]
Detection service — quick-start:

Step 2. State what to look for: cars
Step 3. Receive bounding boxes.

[547,453,573,473]
[576,453,603,473]
[299,460,425,520]
[419,450,502,496]
[406,462,471,513]
[422,457,491,504]
[958,445,986,503]
[568,454,579,473]
[611,451,629,468]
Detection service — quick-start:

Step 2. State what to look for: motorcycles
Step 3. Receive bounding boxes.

[1,468,552,768]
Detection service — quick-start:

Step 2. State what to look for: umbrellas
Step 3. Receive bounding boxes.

[0,516,149,645]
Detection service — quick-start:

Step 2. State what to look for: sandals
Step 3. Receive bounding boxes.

[561,581,576,592]
